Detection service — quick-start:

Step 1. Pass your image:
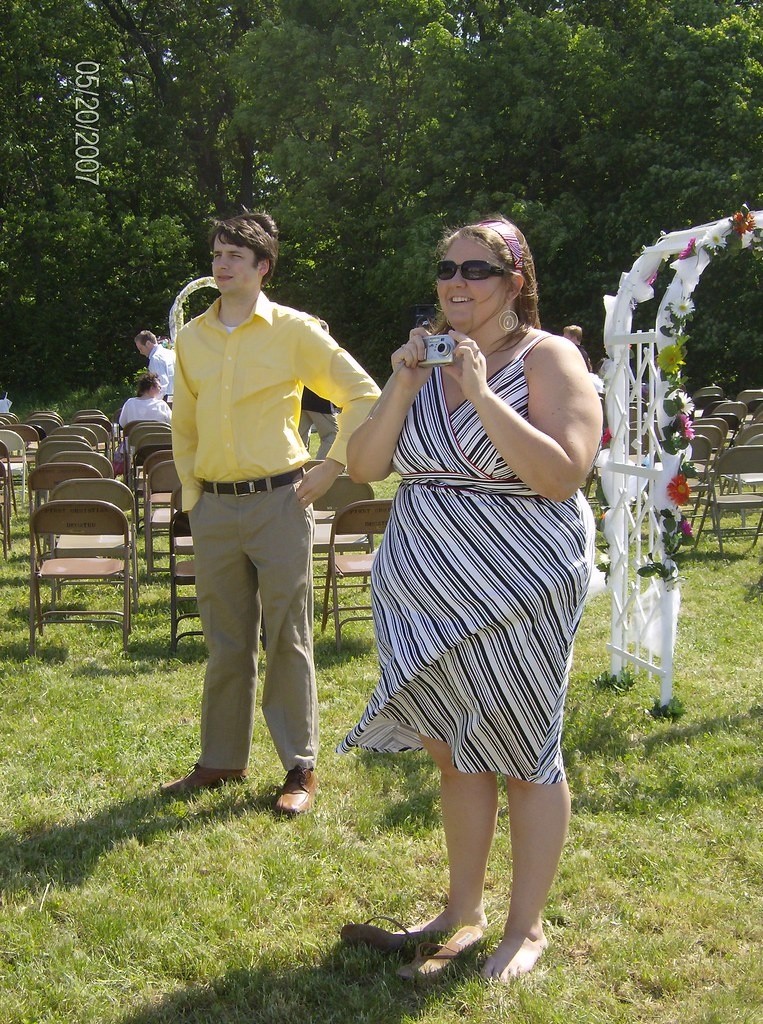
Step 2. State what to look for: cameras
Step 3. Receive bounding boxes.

[417,335,459,367]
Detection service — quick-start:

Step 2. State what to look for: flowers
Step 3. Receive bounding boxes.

[601,205,757,583]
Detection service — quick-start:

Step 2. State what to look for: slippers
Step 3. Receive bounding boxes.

[395,925,482,982]
[341,917,412,949]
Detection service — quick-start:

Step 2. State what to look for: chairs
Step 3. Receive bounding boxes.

[600,385,763,558]
[0,410,393,653]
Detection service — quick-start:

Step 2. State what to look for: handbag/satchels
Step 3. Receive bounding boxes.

[113,441,131,477]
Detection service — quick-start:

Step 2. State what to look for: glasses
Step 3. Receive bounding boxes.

[436,260,509,280]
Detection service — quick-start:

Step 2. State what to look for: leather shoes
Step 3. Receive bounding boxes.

[275,764,317,814]
[162,762,251,798]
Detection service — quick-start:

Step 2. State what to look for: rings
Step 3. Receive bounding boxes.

[401,344,405,349]
[473,348,480,353]
[302,497,306,502]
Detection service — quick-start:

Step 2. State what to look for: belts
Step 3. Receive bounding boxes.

[203,465,303,498]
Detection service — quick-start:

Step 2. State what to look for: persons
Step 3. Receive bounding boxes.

[119,373,172,453]
[563,324,593,373]
[160,213,383,816]
[135,330,176,400]
[347,216,604,982]
